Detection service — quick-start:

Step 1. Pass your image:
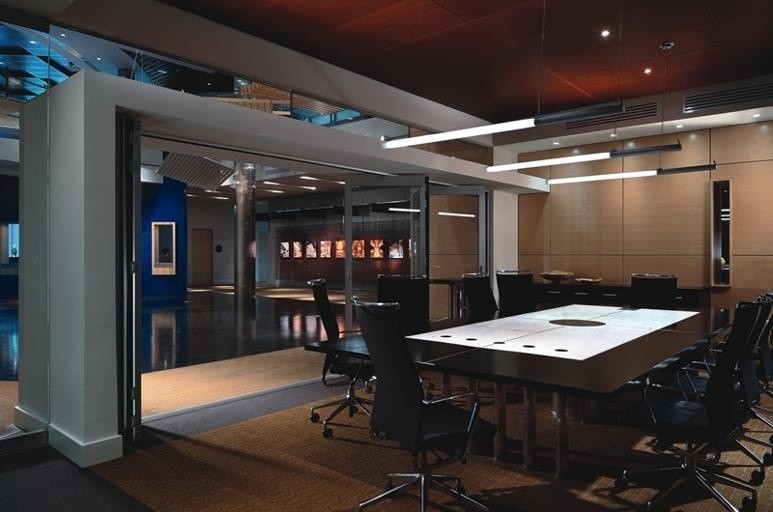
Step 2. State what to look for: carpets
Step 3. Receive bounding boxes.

[187,282,377,305]
[98,379,773,512]
[137,345,333,418]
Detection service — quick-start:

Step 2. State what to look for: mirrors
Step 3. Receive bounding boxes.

[709,178,733,288]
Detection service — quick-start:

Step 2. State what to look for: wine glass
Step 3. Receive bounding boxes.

[539,273,572,284]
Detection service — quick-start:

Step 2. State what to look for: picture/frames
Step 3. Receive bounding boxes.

[151,222,176,276]
